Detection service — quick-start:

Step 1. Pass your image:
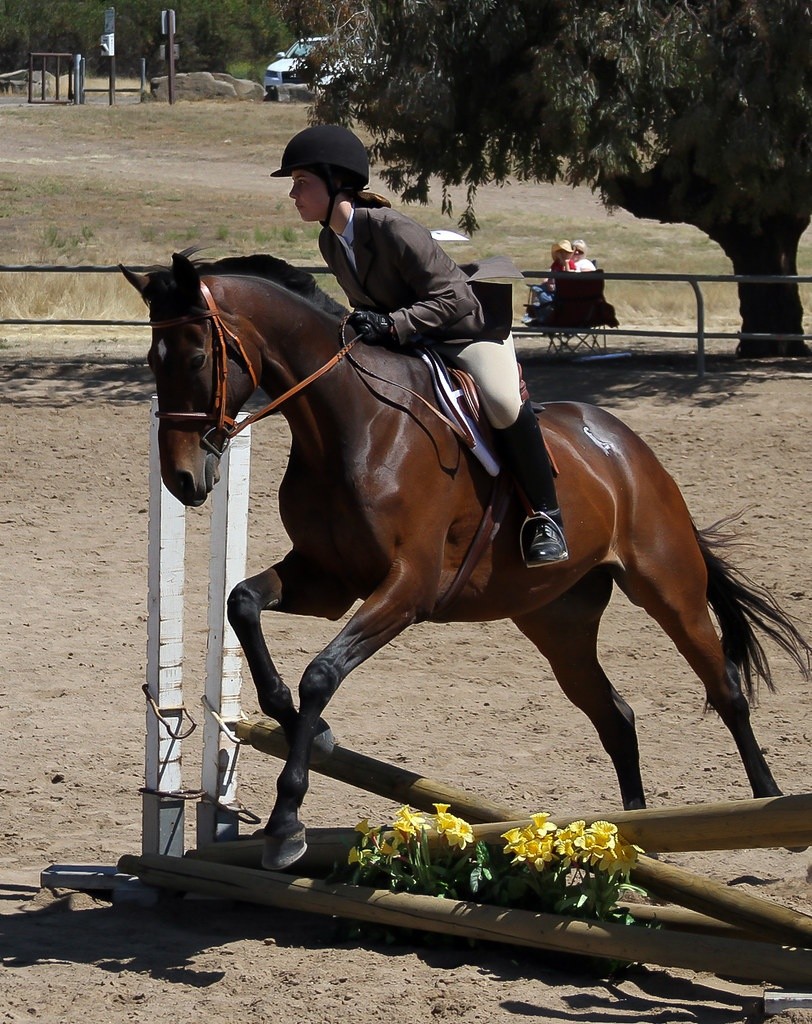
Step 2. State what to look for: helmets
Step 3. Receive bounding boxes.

[269,125,370,184]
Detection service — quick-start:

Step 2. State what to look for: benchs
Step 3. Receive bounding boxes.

[524,267,620,356]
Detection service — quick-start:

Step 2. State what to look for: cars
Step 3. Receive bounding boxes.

[263,38,386,93]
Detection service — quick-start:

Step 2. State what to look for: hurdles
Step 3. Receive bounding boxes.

[115,394,810,987]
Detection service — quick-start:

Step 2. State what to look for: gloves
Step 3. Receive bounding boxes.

[350,310,395,345]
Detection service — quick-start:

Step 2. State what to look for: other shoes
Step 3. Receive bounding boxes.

[521,315,536,323]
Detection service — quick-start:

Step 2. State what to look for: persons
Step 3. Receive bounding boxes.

[267,124,570,570]
[573,239,598,271]
[529,237,574,297]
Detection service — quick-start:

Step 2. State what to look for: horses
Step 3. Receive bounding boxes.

[118,253,812,874]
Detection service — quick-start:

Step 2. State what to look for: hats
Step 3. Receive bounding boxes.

[572,239,586,254]
[551,239,576,260]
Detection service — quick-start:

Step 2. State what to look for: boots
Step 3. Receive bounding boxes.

[492,399,568,562]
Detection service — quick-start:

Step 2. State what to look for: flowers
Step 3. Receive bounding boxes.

[347,802,656,980]
